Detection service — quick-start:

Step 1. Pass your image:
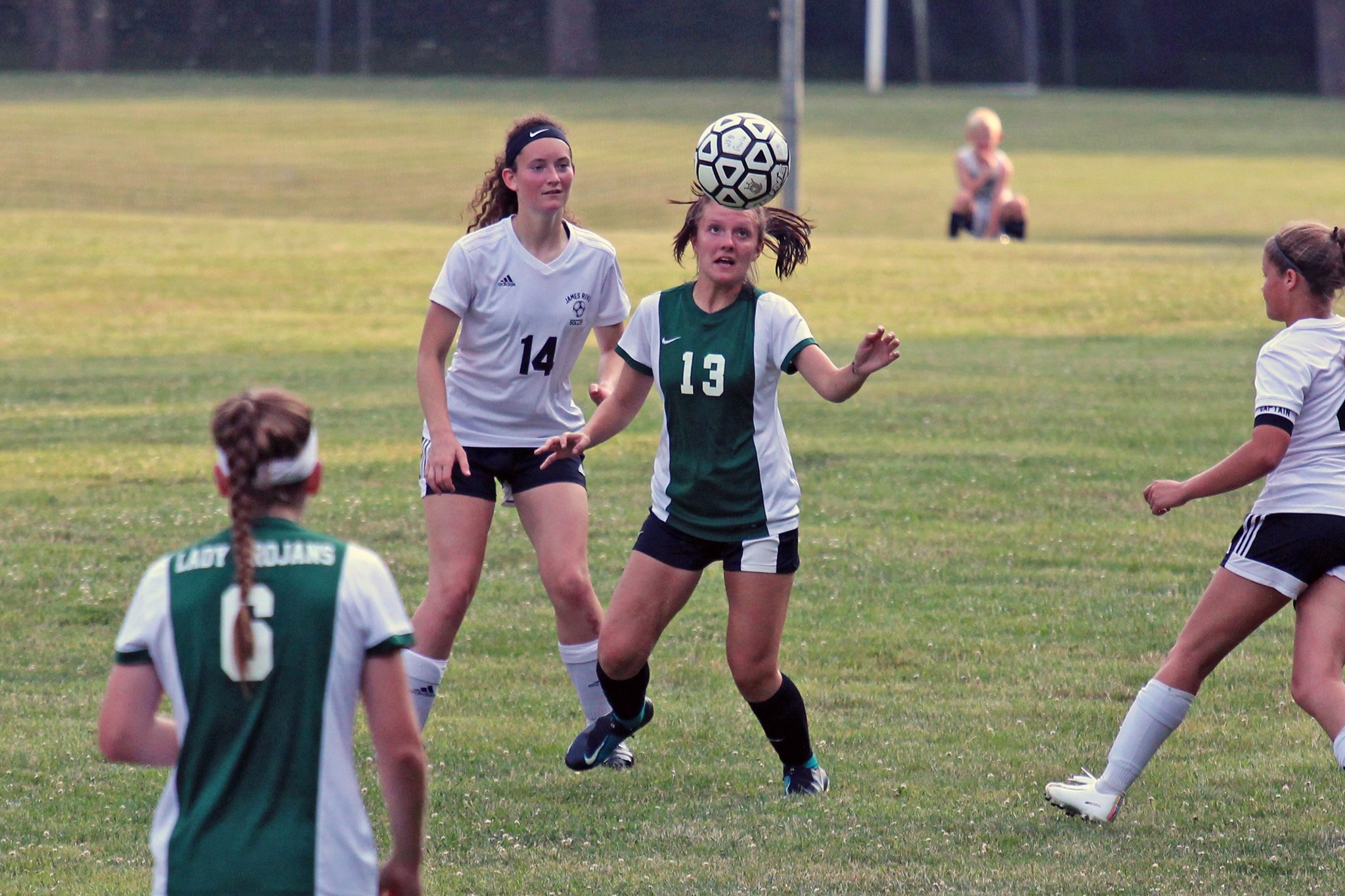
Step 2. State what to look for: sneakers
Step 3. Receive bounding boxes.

[1043,765,1122,827]
[602,742,633,769]
[564,696,654,771]
[783,763,832,801]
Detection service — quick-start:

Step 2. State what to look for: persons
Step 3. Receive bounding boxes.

[401,115,633,767]
[97,391,426,896]
[1042,222,1345,826]
[535,197,899,796]
[947,106,1028,242]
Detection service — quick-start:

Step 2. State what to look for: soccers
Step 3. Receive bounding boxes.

[693,112,791,210]
